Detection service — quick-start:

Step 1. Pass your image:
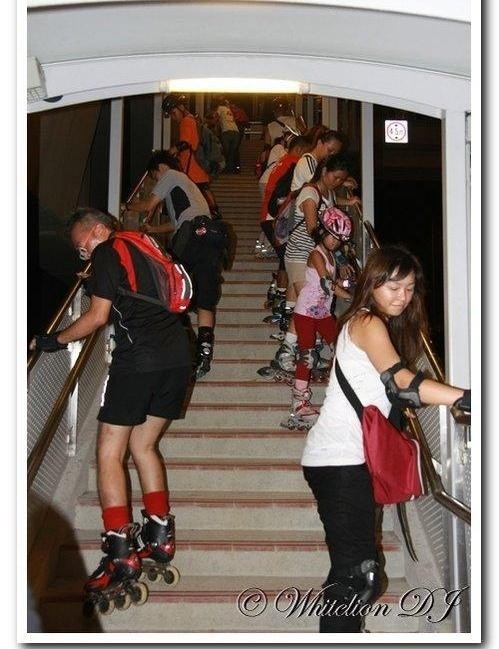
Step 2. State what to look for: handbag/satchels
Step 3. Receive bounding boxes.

[361,406,431,505]
[192,214,235,271]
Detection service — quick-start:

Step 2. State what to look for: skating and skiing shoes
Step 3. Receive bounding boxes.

[197,331,213,378]
[253,240,280,259]
[82,523,148,616]
[137,512,179,587]
[256,272,334,431]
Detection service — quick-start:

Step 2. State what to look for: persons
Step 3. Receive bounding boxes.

[28,91,470,634]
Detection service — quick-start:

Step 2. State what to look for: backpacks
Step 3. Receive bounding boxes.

[106,230,195,313]
[229,104,250,125]
[272,184,322,244]
[254,143,272,179]
[185,117,227,179]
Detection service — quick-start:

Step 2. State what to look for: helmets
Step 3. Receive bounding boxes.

[282,125,302,138]
[162,95,188,118]
[321,208,353,240]
[273,97,289,112]
